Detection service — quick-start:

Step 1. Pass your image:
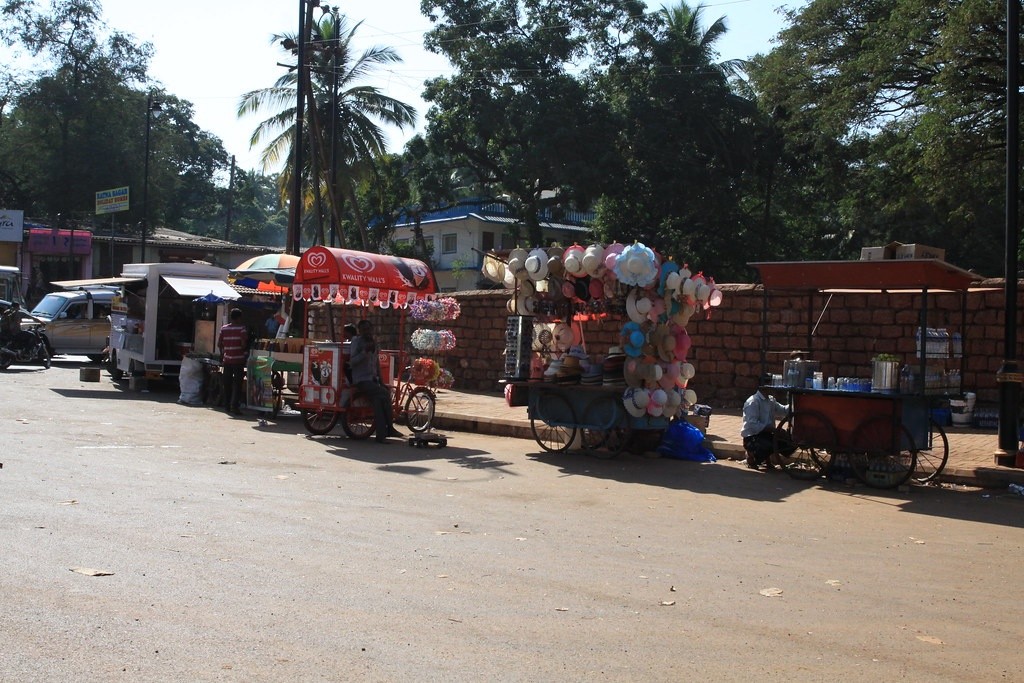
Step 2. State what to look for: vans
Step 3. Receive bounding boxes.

[50,263,242,391]
[20,290,119,362]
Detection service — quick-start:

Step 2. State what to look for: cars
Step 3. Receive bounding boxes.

[0,300,29,317]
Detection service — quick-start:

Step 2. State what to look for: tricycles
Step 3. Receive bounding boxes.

[292,246,437,440]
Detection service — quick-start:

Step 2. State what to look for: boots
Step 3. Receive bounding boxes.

[226,400,243,415]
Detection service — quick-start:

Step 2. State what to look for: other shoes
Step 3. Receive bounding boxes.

[743,463,759,470]
[763,462,776,470]
[377,427,403,444]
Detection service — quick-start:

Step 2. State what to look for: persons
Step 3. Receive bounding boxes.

[218,308,248,417]
[3,300,46,358]
[343,324,357,383]
[350,320,405,444]
[741,372,798,470]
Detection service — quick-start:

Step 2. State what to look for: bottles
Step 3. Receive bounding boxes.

[975,408,999,428]
[925,375,941,388]
[793,363,800,387]
[900,364,914,391]
[916,326,949,358]
[949,369,960,387]
[952,331,963,358]
[787,363,794,386]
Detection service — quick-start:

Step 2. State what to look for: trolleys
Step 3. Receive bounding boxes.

[746,259,985,490]
[472,242,722,458]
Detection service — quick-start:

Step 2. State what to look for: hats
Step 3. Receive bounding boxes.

[482,242,624,317]
[535,323,626,388]
[612,241,723,419]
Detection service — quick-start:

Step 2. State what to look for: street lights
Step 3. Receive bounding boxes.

[142,86,163,263]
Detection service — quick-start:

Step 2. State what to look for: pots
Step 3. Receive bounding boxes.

[782,359,821,387]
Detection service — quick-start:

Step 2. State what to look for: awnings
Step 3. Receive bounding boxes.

[50,277,147,301]
[158,275,242,299]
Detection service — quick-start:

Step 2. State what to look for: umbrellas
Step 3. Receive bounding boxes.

[229,253,303,317]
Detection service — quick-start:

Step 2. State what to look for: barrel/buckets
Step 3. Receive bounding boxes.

[174,341,195,360]
[871,361,899,390]
[949,391,976,427]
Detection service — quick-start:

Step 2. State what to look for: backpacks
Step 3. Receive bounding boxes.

[0,312,17,333]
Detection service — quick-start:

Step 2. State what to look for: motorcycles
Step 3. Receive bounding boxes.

[0,322,50,372]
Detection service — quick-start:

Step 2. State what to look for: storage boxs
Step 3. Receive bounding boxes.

[866,470,912,485]
[860,240,903,260]
[895,244,945,262]
[974,417,998,430]
[80,367,101,382]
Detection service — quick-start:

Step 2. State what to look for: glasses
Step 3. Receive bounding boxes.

[506,318,534,377]
